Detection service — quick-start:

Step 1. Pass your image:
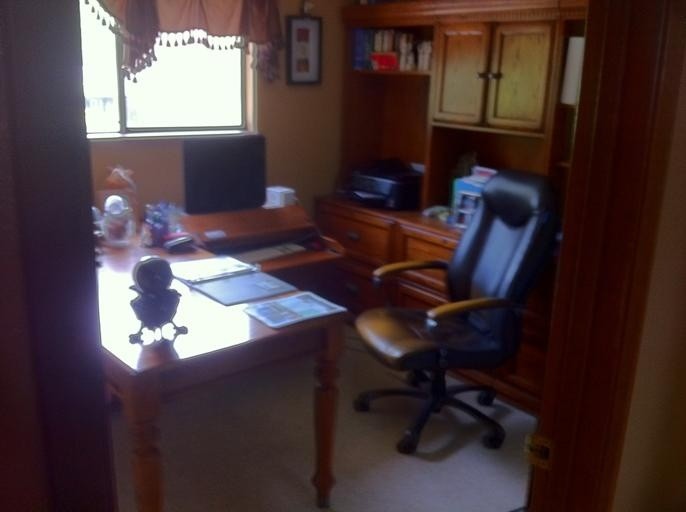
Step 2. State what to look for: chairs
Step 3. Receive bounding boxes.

[354,170,557,454]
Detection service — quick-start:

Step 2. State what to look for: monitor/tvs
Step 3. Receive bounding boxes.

[183,134,265,214]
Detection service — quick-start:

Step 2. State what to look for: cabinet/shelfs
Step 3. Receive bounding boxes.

[395,223,549,408]
[438,29,554,133]
[318,203,390,310]
[428,125,548,235]
[345,24,435,221]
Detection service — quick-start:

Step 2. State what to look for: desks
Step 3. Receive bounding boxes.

[95,201,349,512]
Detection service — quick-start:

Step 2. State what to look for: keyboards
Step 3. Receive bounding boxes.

[231,242,306,263]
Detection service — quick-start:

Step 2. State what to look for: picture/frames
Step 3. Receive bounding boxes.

[285,14,324,85]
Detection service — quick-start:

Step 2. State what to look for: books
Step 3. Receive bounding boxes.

[348,27,432,72]
[243,287,347,331]
[451,167,501,230]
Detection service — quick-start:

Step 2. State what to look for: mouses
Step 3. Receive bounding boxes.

[308,239,325,252]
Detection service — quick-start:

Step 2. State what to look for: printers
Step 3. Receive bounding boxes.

[350,167,421,210]
